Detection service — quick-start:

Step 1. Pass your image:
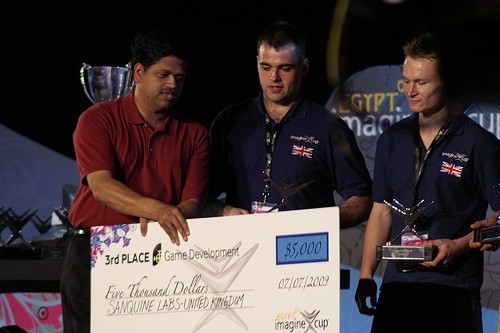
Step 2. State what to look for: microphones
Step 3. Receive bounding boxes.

[473,224,500,251]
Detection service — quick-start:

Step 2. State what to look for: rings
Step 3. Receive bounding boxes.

[168,224,172,228]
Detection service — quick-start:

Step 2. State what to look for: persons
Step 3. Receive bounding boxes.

[354,34,500,333]
[200,24,373,230]
[469,210,500,251]
[60,32,211,333]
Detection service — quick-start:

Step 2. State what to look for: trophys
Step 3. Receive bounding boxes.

[80,62,135,105]
